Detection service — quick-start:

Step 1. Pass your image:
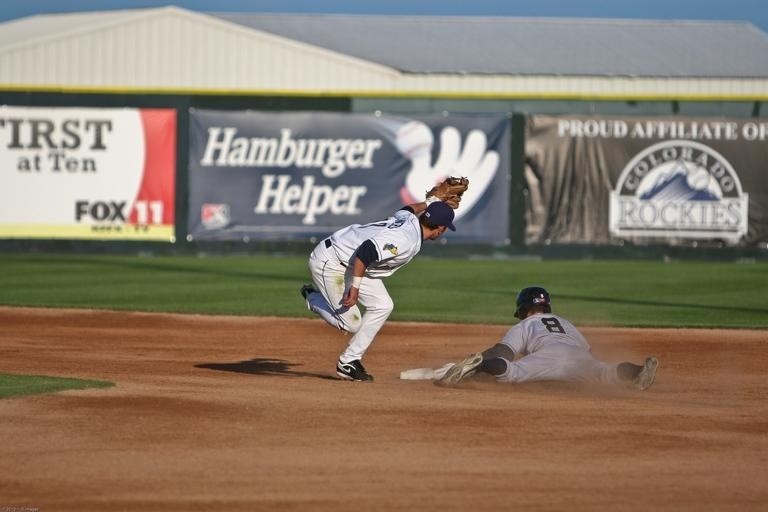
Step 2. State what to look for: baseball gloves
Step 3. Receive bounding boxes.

[426,176,469,208]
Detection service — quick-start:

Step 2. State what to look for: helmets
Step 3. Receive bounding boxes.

[513,287,551,319]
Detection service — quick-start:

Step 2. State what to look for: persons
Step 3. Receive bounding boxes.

[437,287,660,391]
[300,175,470,382]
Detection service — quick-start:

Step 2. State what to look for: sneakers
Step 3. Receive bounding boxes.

[336,358,373,382]
[437,352,484,388]
[299,284,319,300]
[632,357,659,391]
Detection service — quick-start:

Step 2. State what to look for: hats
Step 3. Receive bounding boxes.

[425,202,457,232]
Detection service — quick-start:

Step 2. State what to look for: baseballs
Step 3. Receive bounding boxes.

[687,165,710,191]
